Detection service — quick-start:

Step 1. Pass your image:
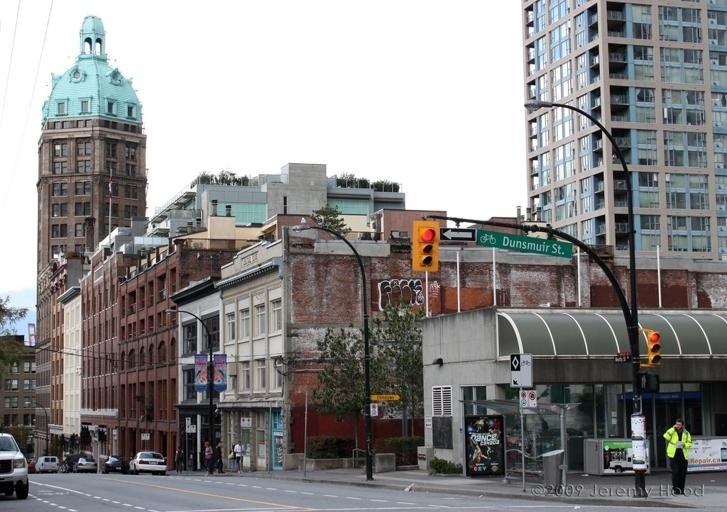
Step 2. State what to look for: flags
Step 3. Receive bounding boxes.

[213,353,228,392]
[194,354,208,392]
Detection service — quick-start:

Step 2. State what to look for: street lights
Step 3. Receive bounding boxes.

[25,401,48,456]
[292,225,372,481]
[165,308,215,474]
[525,99,649,497]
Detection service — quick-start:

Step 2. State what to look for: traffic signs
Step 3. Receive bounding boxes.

[475,230,573,259]
[370,394,400,401]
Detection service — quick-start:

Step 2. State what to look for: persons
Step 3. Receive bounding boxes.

[661,418,693,495]
[174,440,244,475]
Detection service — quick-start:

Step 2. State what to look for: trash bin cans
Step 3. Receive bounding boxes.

[541,449,565,488]
[228,457,237,472]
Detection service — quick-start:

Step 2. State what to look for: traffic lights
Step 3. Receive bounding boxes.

[412,220,440,273]
[646,330,661,366]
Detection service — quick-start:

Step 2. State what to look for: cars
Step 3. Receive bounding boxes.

[0,433,35,499]
[76,457,97,472]
[129,451,167,475]
[104,455,125,473]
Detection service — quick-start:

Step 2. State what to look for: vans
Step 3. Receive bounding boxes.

[36,456,59,472]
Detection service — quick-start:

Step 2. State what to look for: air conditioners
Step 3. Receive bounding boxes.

[577,3,590,235]
[708,0,727,253]
